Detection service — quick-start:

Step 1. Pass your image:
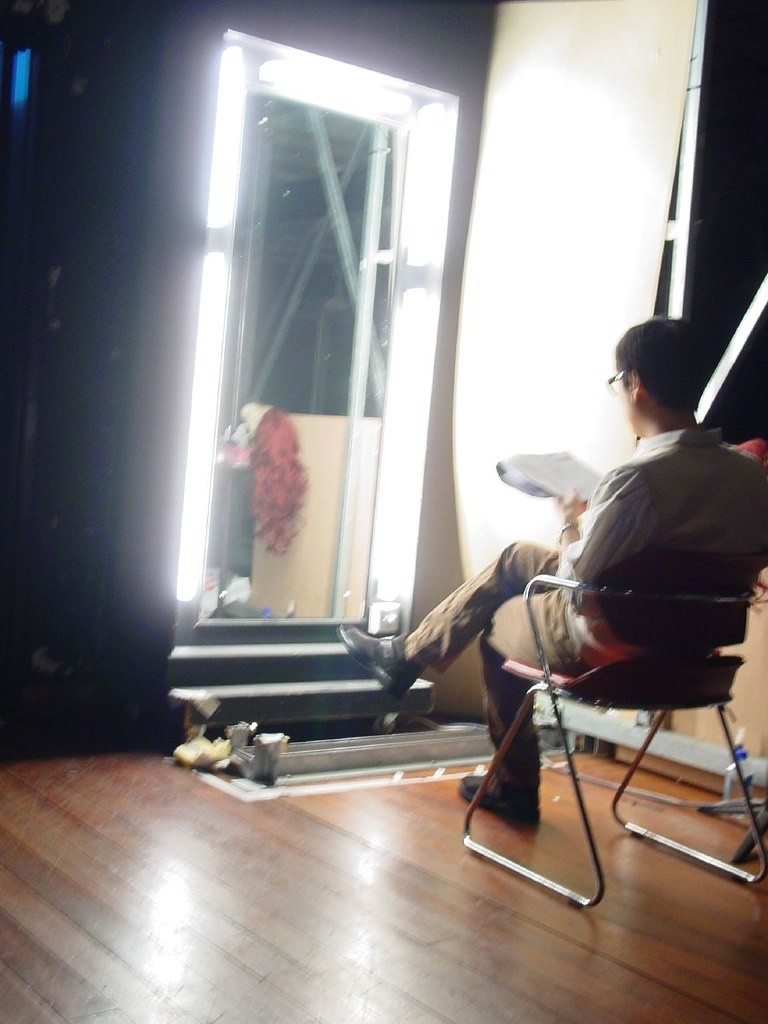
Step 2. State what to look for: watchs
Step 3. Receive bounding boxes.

[556,520,578,545]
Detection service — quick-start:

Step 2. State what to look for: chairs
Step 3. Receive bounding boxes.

[459,575,768,909]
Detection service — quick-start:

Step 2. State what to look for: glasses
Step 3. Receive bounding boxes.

[607,370,627,398]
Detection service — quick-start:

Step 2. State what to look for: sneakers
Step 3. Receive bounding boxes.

[336,622,420,702]
[459,774,541,825]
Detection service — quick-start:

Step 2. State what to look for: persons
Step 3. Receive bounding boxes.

[334,316,768,826]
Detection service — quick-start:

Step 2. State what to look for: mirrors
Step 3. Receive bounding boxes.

[173,26,460,630]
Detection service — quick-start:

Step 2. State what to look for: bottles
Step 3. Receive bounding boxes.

[725,750,754,818]
